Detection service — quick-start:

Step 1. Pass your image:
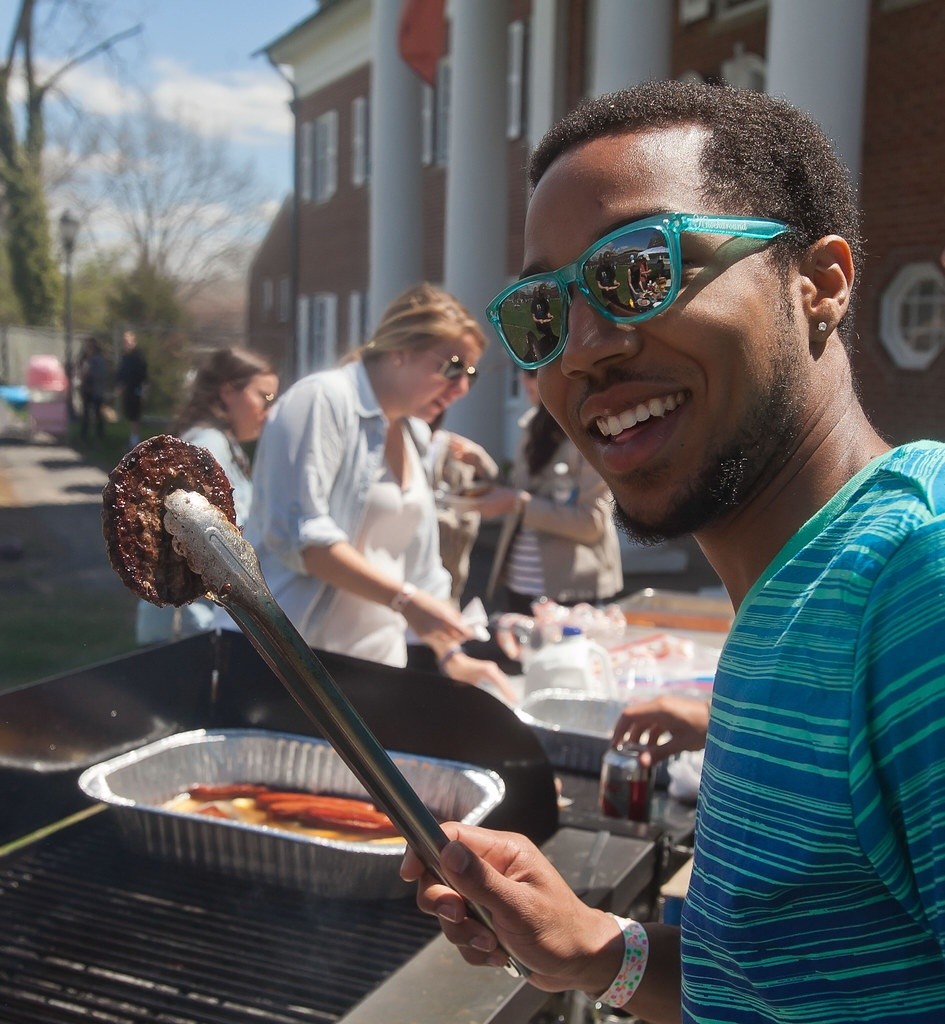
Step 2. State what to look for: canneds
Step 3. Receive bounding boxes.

[597,742,655,825]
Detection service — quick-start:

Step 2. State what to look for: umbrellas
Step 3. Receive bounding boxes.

[638,245,670,260]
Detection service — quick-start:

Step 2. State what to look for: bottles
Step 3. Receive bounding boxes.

[557,627,588,663]
[551,463,576,506]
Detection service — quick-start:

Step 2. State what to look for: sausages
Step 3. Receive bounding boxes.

[185,784,400,834]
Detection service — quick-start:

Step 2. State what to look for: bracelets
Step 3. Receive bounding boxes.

[391,582,417,614]
[435,643,464,670]
[582,911,649,1011]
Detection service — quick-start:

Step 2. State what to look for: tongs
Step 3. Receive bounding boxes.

[163,491,531,981]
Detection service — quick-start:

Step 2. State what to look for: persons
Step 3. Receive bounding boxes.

[595,251,652,310]
[530,284,556,337]
[399,81,945,1024]
[118,330,148,448]
[75,337,110,441]
[243,281,520,714]
[134,347,279,648]
[448,371,623,617]
[418,411,480,601]
[611,694,711,765]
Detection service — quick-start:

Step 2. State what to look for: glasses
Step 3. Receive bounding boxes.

[484,208,801,374]
[423,342,482,389]
[243,380,277,412]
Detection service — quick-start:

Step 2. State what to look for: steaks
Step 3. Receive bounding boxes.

[101,436,237,609]
